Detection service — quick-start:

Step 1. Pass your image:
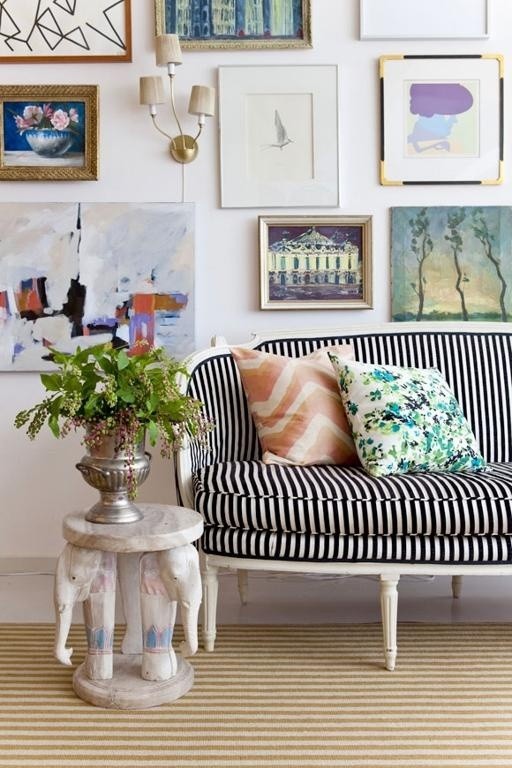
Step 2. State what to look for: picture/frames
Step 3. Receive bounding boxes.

[258,214,374,310]
[0,84,100,181]
[378,52,505,187]
[154,0,313,52]
[359,0,490,41]
[217,64,340,207]
[0,0,132,65]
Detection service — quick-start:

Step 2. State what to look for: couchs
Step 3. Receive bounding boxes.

[172,331,512,670]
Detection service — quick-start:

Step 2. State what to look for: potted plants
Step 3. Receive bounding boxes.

[13,337,220,526]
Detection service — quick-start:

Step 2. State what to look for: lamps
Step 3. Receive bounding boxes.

[140,33,216,164]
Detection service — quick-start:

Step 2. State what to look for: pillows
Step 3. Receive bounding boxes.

[228,345,356,468]
[327,352,493,478]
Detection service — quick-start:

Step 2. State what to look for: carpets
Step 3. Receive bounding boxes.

[2,621,512,768]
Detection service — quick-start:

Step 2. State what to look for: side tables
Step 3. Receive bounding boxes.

[55,501,206,711]
